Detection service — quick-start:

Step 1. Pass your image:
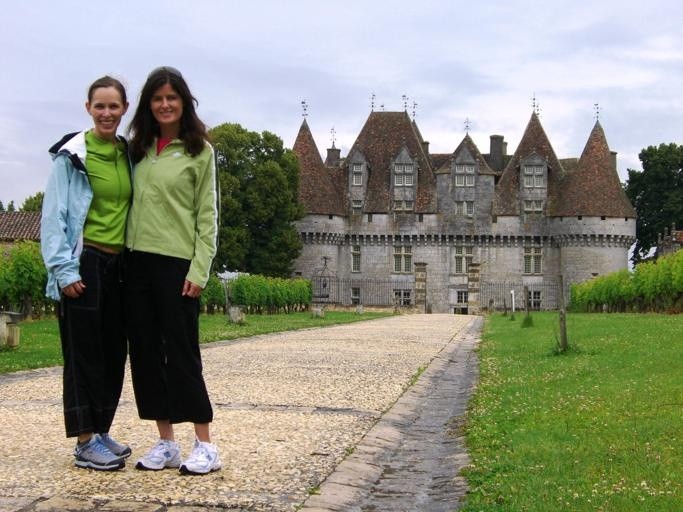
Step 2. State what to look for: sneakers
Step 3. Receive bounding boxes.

[72,433,131,459]
[178,441,221,474]
[134,439,182,471]
[73,438,125,470]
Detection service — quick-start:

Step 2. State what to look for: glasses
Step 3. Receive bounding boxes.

[147,66,182,79]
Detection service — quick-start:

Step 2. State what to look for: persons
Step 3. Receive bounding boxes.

[37,75,138,470]
[125,65,225,474]
